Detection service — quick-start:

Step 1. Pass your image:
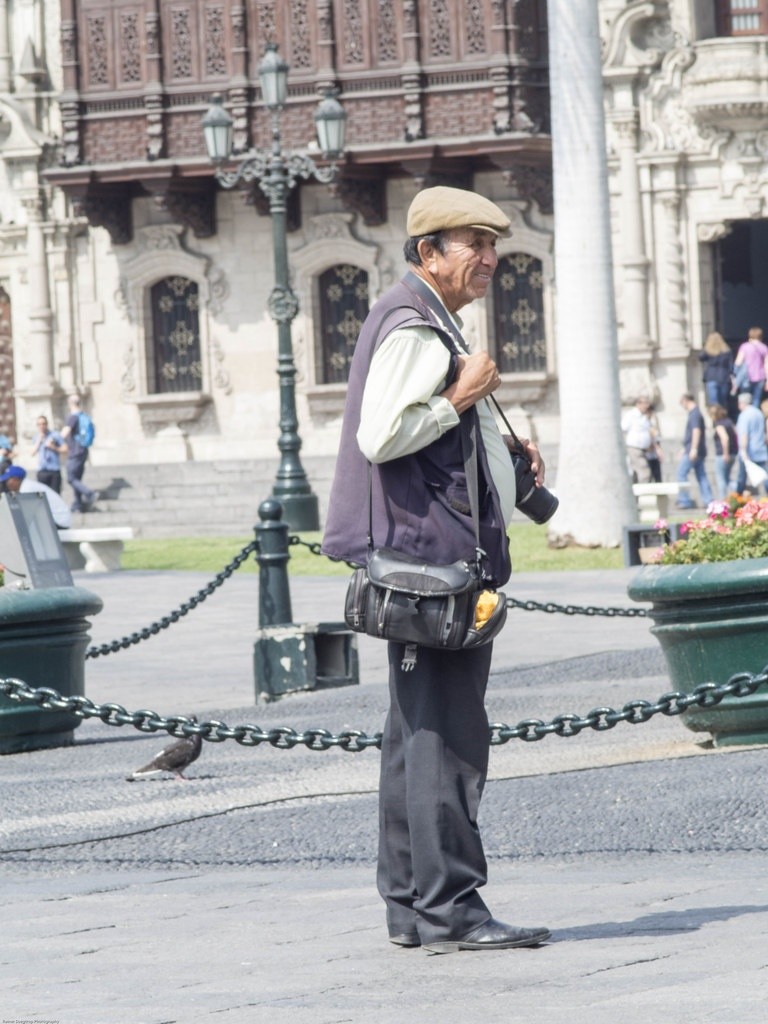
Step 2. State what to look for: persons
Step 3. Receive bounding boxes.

[0,466,72,529]
[622,397,664,484]
[677,328,768,510]
[29,416,69,494]
[320,185,554,956]
[0,421,12,493]
[61,395,99,513]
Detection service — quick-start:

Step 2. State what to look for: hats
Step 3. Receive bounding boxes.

[0,465,26,481]
[406,185,514,238]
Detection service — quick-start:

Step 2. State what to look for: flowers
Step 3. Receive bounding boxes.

[652,492,768,565]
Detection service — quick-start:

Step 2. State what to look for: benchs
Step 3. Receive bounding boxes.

[57,528,138,572]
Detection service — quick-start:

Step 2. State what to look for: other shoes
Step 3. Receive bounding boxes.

[71,506,84,513]
[86,491,99,507]
[676,501,694,508]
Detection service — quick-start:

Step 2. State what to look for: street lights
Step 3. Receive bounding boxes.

[197,54,348,531]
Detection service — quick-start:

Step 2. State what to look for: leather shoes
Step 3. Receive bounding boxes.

[389,929,421,946]
[422,917,552,954]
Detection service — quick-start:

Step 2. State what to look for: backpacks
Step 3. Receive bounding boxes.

[72,412,95,448]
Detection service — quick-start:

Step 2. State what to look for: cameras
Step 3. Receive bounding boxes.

[510,452,559,525]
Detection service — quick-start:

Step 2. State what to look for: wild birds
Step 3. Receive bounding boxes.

[123,715,203,782]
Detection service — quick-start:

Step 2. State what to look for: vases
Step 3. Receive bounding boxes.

[0,587,103,756]
[627,557,768,747]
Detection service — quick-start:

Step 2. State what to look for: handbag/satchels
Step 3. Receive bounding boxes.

[345,548,507,670]
[743,457,768,487]
